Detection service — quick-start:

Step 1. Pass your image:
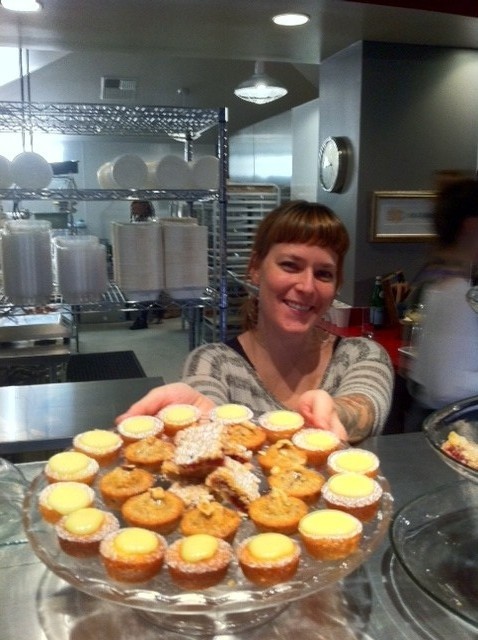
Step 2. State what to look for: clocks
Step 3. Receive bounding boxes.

[317,136,348,193]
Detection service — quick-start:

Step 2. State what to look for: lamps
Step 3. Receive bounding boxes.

[234,62,288,104]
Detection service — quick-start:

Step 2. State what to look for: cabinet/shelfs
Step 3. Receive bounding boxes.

[168,182,282,349]
[1,101,230,356]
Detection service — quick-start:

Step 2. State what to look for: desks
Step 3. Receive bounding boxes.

[0,376,166,455]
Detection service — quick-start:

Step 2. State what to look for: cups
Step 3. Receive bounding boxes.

[331,302,346,325]
[336,305,352,328]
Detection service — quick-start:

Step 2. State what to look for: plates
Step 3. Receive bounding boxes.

[422,394,478,484]
[144,154,189,189]
[111,219,164,302]
[189,155,220,190]
[381,545,478,639]
[0,155,12,189]
[0,218,53,307]
[388,478,478,636]
[160,216,209,301]
[11,150,53,191]
[51,234,108,304]
[96,152,149,190]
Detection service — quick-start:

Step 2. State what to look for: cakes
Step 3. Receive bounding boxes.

[238,532,301,588]
[321,472,382,523]
[158,403,202,434]
[249,491,309,533]
[54,507,120,557]
[208,403,253,425]
[100,464,154,505]
[204,457,260,509]
[120,488,183,535]
[166,479,203,507]
[224,423,267,463]
[126,440,174,468]
[330,448,378,482]
[38,482,96,526]
[298,509,363,560]
[115,415,164,442]
[268,465,326,506]
[175,425,223,474]
[260,442,306,474]
[180,500,240,542]
[164,535,234,587]
[292,427,345,461]
[44,453,100,485]
[100,528,165,581]
[70,429,125,466]
[258,411,304,445]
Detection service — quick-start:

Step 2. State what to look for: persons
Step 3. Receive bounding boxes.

[128,199,168,331]
[399,178,477,433]
[112,198,395,448]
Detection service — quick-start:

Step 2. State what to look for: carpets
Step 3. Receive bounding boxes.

[0,349,148,464]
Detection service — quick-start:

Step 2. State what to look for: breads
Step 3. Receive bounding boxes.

[439,431,478,471]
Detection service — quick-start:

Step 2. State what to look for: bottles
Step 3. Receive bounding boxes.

[370,275,386,330]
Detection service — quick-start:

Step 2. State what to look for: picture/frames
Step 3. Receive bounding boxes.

[368,191,440,244]
